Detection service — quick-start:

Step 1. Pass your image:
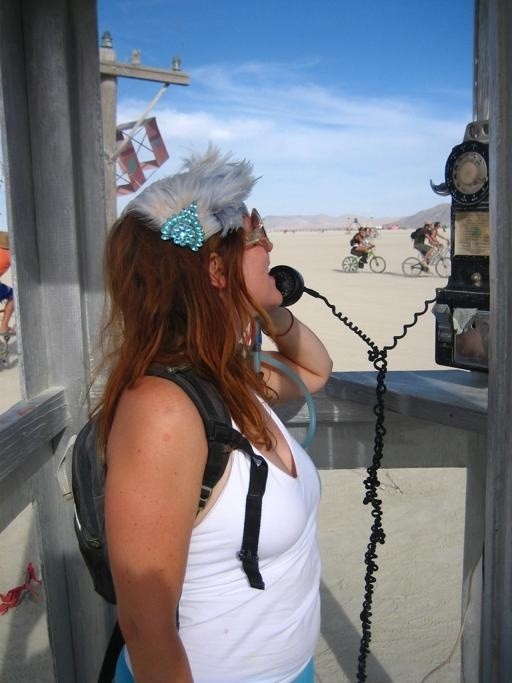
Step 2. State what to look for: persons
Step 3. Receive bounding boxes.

[349,220,451,275]
[0,230,16,336]
[54,137,335,683]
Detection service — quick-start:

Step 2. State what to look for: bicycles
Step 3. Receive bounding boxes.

[342,244,386,273]
[402,239,451,278]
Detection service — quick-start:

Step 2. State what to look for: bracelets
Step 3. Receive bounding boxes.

[259,303,296,339]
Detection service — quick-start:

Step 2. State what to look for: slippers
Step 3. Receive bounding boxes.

[0,328,16,335]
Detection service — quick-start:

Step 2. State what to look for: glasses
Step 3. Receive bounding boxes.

[237,206,273,251]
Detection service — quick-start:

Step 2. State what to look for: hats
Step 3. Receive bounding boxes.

[0,232,9,249]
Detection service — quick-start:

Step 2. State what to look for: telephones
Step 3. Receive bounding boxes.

[269,264,305,309]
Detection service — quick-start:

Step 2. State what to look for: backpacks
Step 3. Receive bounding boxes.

[74,356,229,603]
[410,227,424,238]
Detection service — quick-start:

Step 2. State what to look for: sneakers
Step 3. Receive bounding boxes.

[423,259,432,271]
[358,260,368,268]
